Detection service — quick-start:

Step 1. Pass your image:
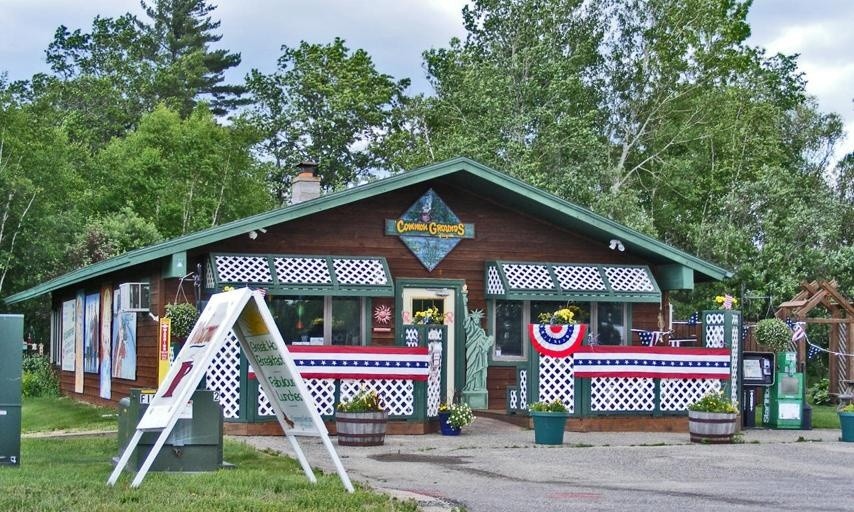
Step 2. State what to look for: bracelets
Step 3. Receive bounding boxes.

[464,304,468,306]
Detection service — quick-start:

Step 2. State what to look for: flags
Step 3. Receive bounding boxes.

[688,311,699,329]
[786,318,805,343]
[638,331,659,346]
[807,345,821,360]
[741,324,749,340]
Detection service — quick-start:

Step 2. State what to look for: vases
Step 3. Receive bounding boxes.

[688,411,737,443]
[438,411,461,436]
[335,410,389,446]
[531,412,568,445]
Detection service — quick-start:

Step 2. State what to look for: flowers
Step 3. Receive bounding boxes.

[336,385,381,412]
[690,391,739,414]
[538,305,589,324]
[413,305,445,325]
[439,401,476,431]
[529,399,568,412]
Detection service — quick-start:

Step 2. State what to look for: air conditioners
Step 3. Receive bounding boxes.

[119,282,158,322]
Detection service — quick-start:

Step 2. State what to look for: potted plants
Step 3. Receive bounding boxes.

[838,404,854,442]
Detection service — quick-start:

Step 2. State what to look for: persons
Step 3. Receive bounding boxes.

[461,292,488,391]
